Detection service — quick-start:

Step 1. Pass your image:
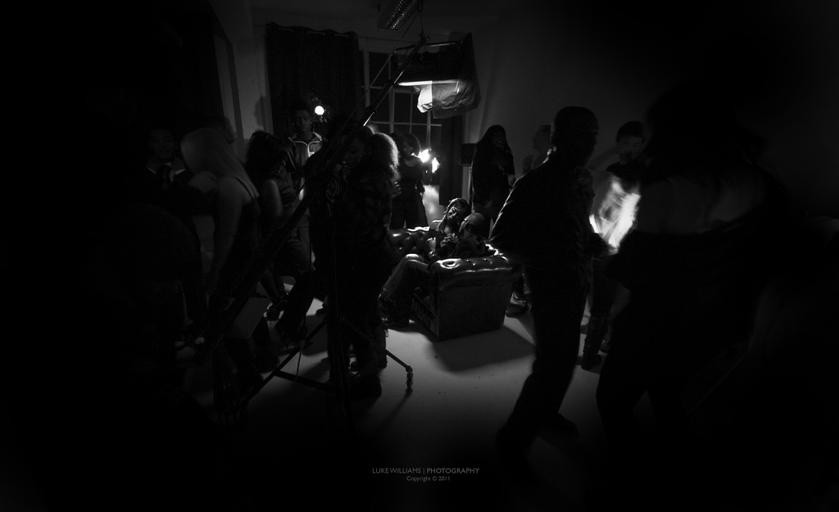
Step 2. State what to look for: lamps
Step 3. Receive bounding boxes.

[390,0,464,87]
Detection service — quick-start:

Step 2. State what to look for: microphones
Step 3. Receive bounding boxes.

[395,41,456,51]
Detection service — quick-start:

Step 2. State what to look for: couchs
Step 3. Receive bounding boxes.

[382,222,524,342]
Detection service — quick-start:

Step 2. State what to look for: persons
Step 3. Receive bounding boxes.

[132,77,836,510]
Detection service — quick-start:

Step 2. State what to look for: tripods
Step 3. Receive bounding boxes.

[242,50,436,420]
